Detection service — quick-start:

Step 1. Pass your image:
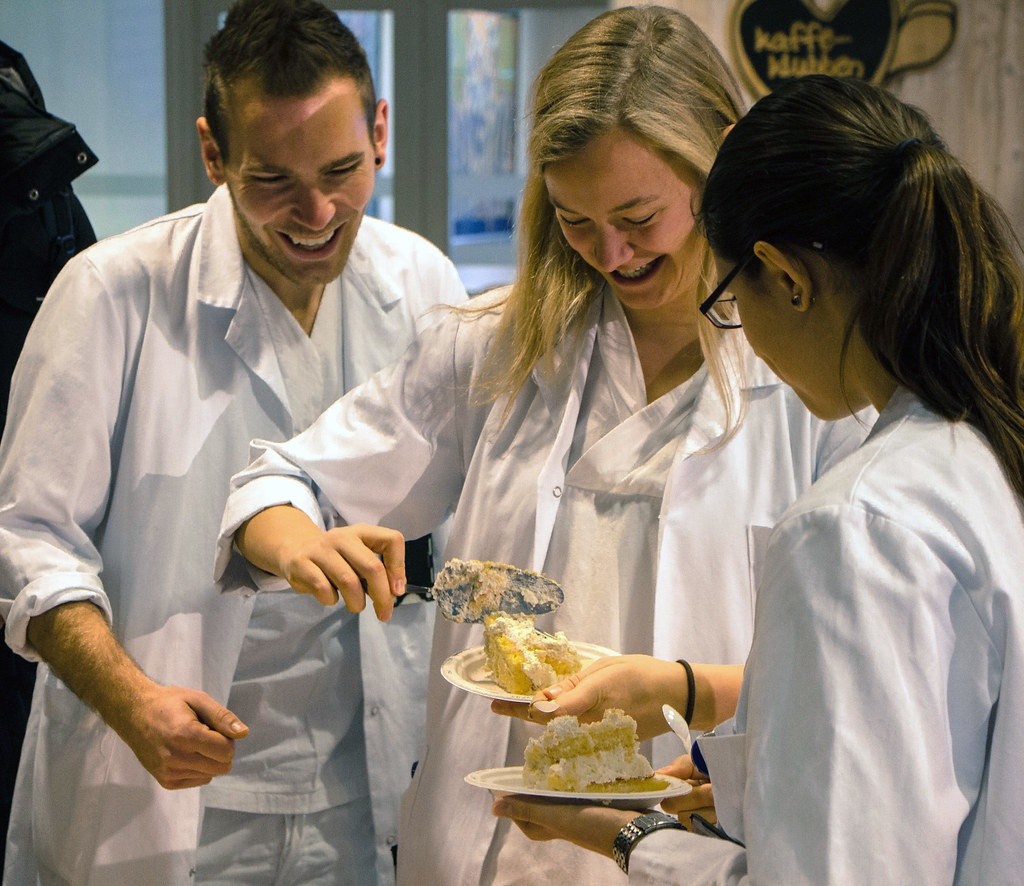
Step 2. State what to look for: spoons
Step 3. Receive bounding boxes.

[662,703,692,762]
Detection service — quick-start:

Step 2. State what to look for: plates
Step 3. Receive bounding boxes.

[440,641,622,703]
[464,766,693,810]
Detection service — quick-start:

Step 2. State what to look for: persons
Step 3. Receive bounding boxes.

[491,73,1024,885]
[213,7,880,886]
[0,0,471,886]
[0,39,99,886]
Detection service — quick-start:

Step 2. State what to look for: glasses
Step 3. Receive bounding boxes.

[697,233,826,330]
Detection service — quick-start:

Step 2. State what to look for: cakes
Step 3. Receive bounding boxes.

[483,610,582,694]
[522,708,671,793]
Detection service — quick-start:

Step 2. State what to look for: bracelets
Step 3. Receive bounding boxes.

[676,659,695,727]
[613,812,688,876]
